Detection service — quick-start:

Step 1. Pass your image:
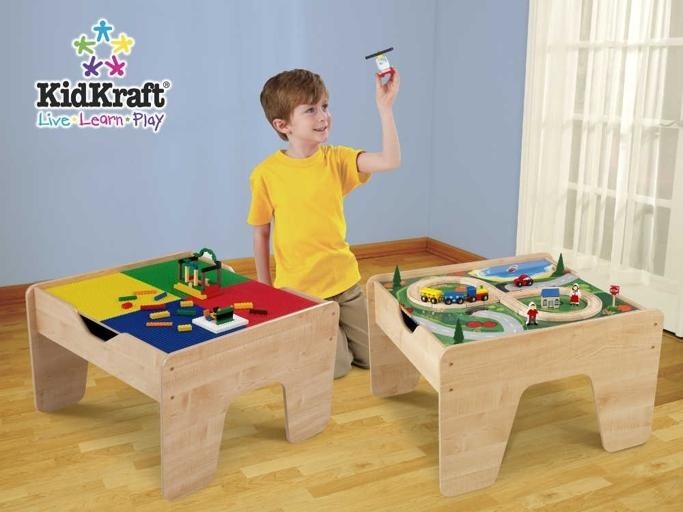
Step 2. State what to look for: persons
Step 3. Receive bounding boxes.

[246,66,402,380]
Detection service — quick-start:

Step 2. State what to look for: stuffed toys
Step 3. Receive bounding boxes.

[118,247,269,335]
[364,47,395,78]
[406,274,603,326]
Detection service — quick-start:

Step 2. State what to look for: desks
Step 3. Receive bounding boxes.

[17,247,343,503]
[363,247,666,501]
